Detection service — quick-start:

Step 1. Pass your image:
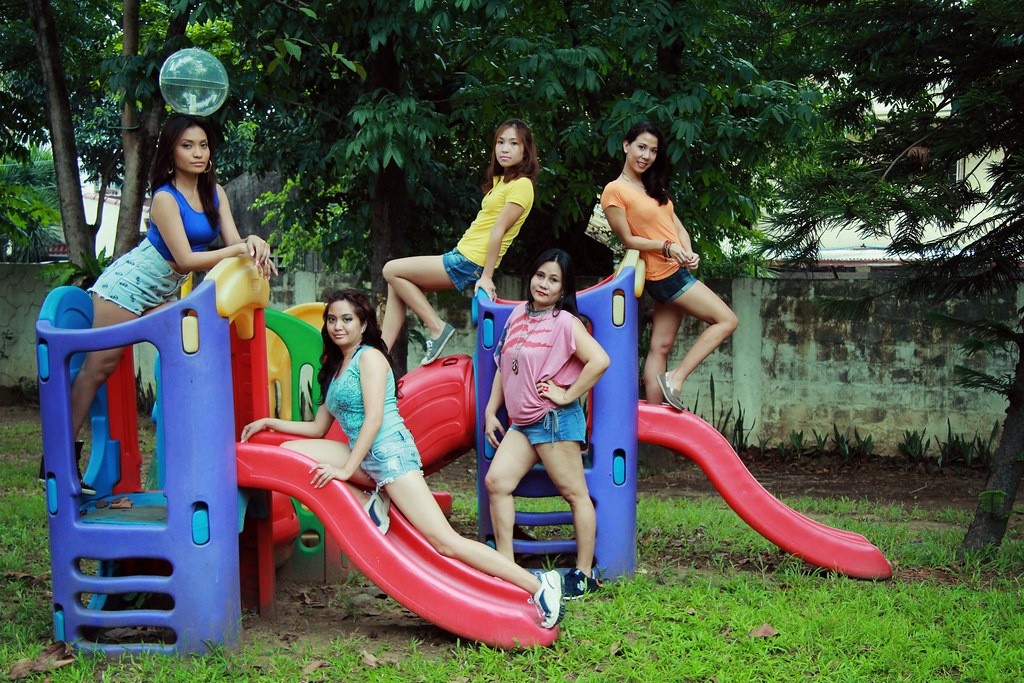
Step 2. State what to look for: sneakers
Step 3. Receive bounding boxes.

[563,568,599,600]
[363,490,390,535]
[421,323,455,365]
[527,570,565,628]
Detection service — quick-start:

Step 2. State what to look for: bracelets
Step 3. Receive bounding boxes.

[661,240,675,258]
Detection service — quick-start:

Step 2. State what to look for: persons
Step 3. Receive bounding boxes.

[38,113,279,494]
[240,288,564,630]
[602,122,739,411]
[484,248,611,599]
[380,119,537,365]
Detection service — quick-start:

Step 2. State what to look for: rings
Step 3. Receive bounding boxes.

[542,386,548,393]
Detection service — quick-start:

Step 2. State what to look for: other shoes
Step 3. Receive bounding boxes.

[656,372,685,411]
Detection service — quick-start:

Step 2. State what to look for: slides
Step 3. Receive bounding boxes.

[236,430,560,651]
[637,398,894,583]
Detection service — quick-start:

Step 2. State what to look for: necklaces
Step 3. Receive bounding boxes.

[621,171,643,188]
[512,304,553,375]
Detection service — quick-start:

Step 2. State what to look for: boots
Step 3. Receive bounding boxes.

[39,441,97,494]
